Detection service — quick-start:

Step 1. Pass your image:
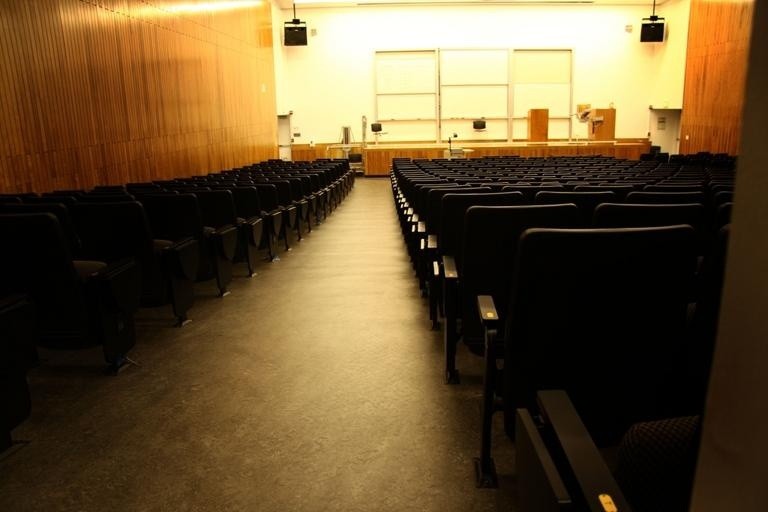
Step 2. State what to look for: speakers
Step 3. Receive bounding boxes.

[371,123,382,132]
[283,27,307,46]
[640,23,664,42]
[473,121,486,129]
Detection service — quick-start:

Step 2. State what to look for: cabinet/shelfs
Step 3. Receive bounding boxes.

[291,143,451,178]
